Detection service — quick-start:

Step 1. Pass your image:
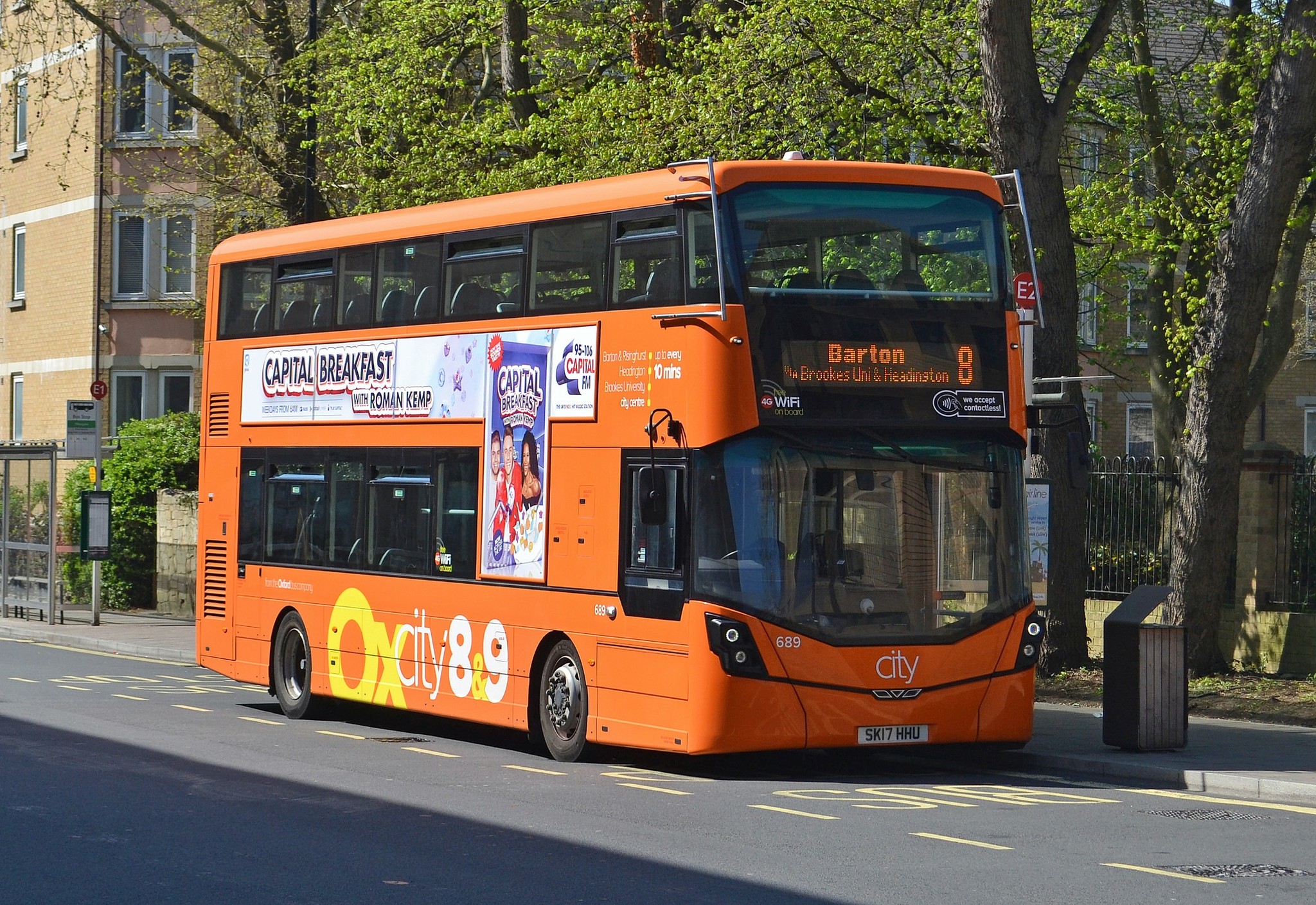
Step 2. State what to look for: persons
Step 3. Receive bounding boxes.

[743,539,796,629]
[488,426,541,543]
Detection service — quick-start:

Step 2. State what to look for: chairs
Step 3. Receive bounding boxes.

[253,260,929,331]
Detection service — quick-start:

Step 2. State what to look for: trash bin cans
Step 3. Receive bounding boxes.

[1103,585,1188,751]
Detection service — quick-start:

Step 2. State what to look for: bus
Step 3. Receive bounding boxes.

[196,149,1092,765]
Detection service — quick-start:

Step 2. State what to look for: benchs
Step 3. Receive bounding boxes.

[12,576,69,624]
